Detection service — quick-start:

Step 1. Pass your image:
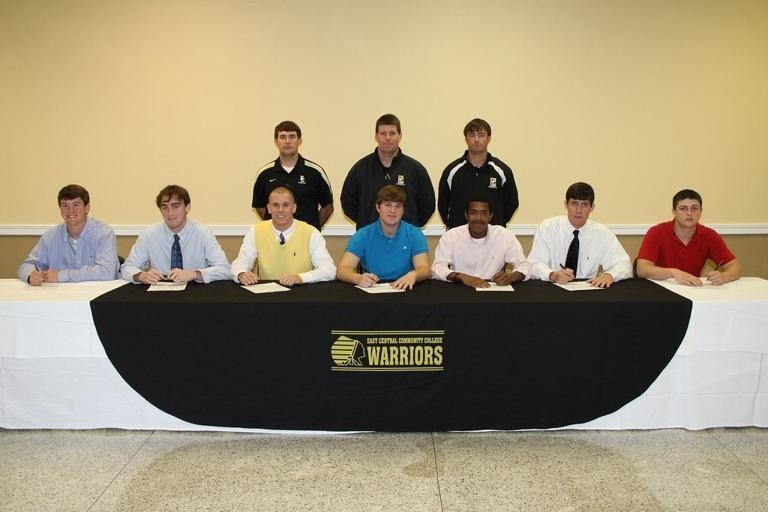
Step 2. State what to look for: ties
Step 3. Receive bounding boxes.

[171,235,183,270]
[565,231,579,279]
[280,233,285,244]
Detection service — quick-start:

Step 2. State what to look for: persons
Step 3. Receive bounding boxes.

[438,117,519,229]
[230,187,337,287]
[251,121,334,236]
[337,184,433,291]
[120,186,233,286]
[636,189,742,287]
[525,182,634,289]
[340,114,436,229]
[17,184,120,286]
[432,198,532,289]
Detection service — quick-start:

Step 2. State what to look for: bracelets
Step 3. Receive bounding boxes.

[453,273,460,281]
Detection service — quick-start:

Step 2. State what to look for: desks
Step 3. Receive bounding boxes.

[1,278,767,434]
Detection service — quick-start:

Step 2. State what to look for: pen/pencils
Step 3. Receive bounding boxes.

[33,260,40,272]
[707,260,724,281]
[560,263,576,281]
[359,266,371,274]
